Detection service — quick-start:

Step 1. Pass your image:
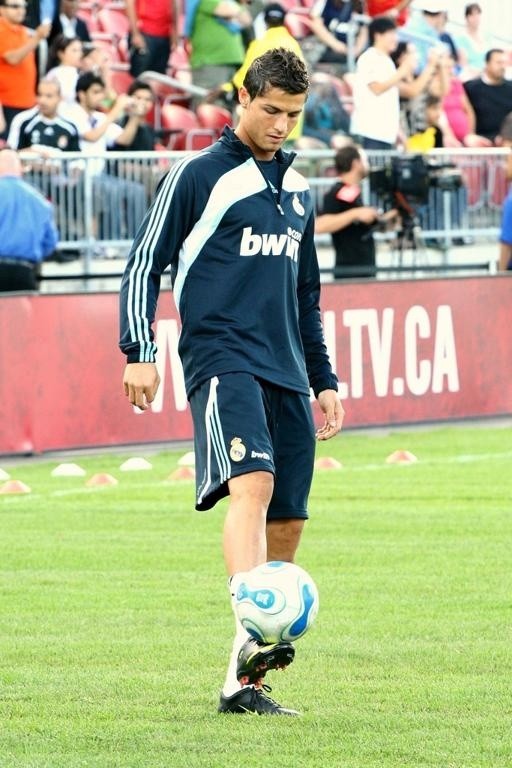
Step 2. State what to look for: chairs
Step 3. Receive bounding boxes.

[60,1,511,217]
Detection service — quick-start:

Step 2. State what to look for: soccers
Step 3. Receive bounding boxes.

[236,560,319,642]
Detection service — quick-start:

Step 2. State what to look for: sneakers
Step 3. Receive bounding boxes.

[236,642,293,684]
[219,688,297,714]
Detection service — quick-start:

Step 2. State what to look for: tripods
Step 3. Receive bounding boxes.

[392,224,434,278]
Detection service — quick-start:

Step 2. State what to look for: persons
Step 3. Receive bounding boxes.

[119,49,346,716]
